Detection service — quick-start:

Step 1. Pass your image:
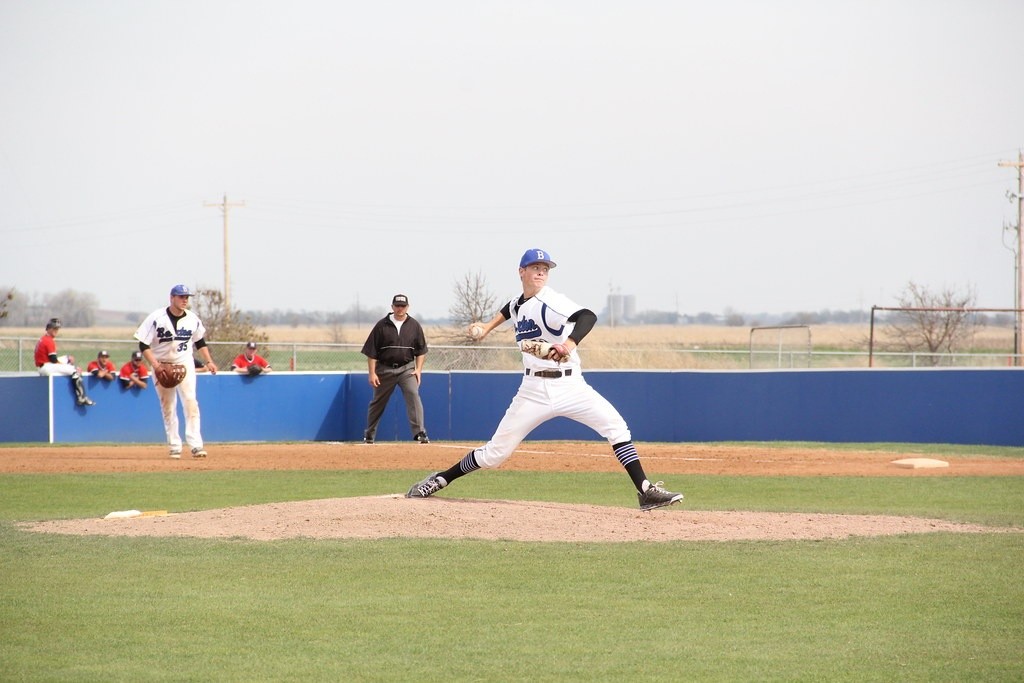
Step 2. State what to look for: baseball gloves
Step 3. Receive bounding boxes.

[520,339,571,364]
[154,361,187,388]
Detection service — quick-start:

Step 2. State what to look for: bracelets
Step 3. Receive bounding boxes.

[206,361,213,364]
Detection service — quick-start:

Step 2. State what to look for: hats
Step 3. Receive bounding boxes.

[393,295,408,305]
[520,249,556,268]
[99,350,109,357]
[49,319,61,327]
[132,351,142,359]
[248,342,256,349]
[171,285,194,296]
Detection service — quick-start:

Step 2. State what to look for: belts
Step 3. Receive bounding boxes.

[380,361,404,369]
[526,369,572,378]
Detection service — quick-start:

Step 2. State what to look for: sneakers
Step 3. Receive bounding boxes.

[192,447,207,457]
[639,484,683,511]
[170,450,181,458]
[418,432,429,443]
[77,397,93,405]
[405,473,443,498]
[366,436,374,443]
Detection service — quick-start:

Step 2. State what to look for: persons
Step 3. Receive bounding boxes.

[134,285,218,458]
[119,351,148,390]
[87,351,115,381]
[360,294,432,444]
[34,318,96,406]
[406,248,683,512]
[231,342,272,373]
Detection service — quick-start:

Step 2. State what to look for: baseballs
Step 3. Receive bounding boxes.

[470,325,485,337]
